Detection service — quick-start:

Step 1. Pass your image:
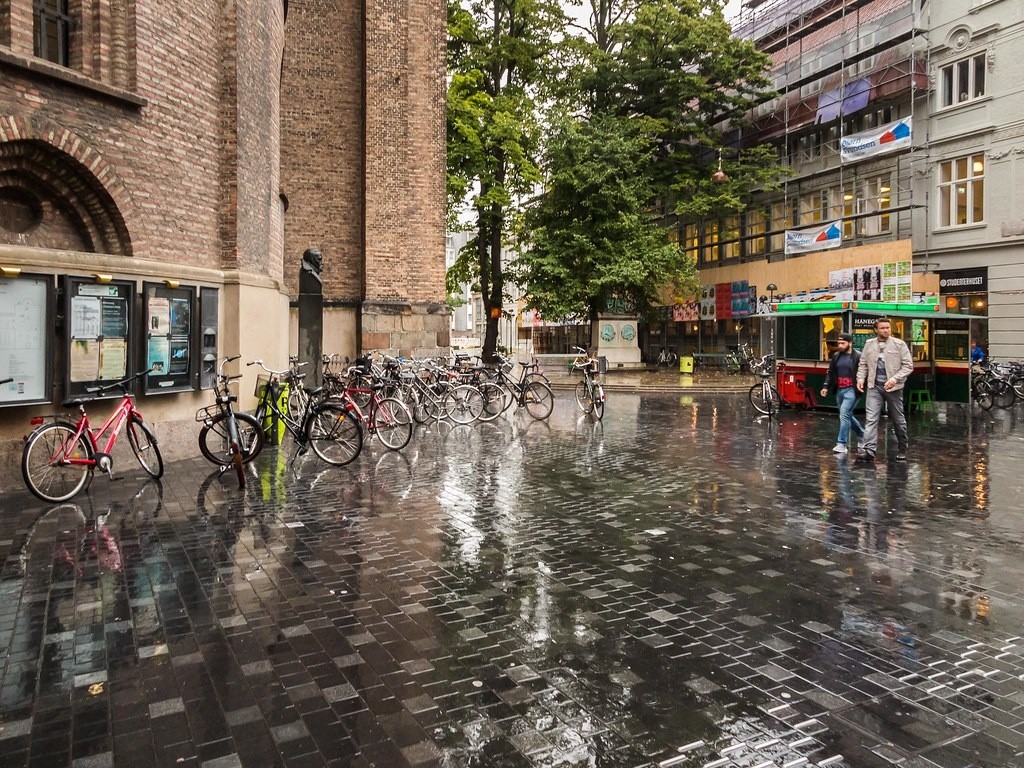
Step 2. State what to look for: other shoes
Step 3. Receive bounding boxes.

[896,448,907,459]
[832,444,848,452]
[855,450,875,461]
[857,437,864,448]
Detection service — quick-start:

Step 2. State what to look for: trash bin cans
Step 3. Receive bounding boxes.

[596,356,607,374]
[776,370,817,411]
[679,356,694,374]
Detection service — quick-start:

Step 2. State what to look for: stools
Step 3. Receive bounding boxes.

[908,389,936,415]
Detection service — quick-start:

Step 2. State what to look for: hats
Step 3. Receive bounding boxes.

[838,333,855,344]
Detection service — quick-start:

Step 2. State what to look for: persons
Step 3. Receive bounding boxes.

[826,318,842,359]
[971,338,985,401]
[820,333,868,452]
[854,266,881,300]
[851,318,913,462]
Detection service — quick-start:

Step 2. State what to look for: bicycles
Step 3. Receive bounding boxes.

[247,350,556,466]
[723,343,765,375]
[570,343,606,420]
[196,355,266,487]
[22,368,165,503]
[748,353,783,422]
[654,345,677,366]
[970,360,1024,410]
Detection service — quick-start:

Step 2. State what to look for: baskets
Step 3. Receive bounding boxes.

[499,359,514,373]
[254,374,287,401]
[749,359,763,373]
[413,363,435,378]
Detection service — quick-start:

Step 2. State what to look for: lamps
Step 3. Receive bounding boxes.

[712,148,726,182]
